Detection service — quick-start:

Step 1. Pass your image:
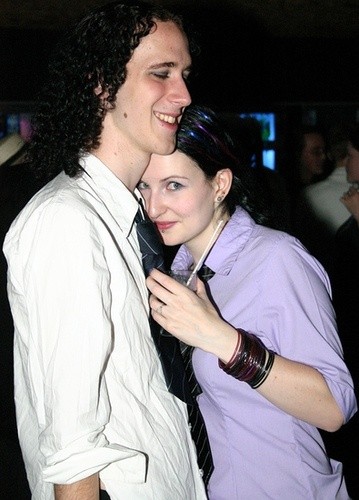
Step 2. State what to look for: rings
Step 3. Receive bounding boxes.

[160,304,164,316]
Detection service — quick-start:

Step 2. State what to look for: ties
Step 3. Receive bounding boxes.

[134,208,214,488]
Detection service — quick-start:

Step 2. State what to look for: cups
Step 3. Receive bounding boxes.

[160,271,197,337]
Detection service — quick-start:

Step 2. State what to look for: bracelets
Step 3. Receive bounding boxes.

[218,328,275,389]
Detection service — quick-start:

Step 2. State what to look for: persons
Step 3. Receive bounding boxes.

[137,106,357,500]
[334,133,359,397]
[272,129,335,299]
[230,119,271,223]
[2,6,209,500]
